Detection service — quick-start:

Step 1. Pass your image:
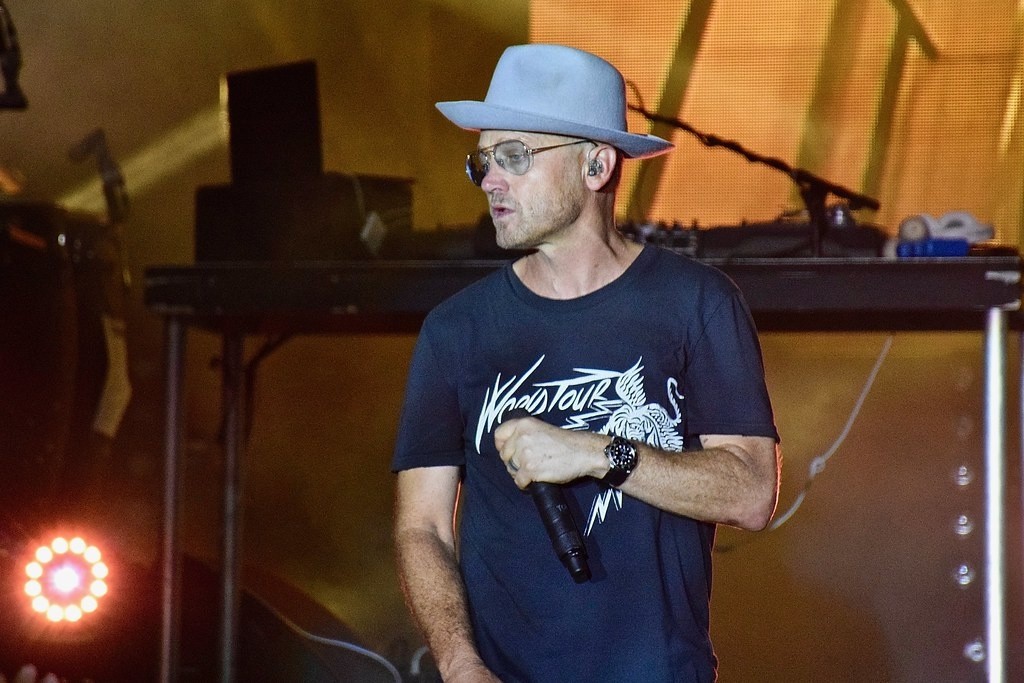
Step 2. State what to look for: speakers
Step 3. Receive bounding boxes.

[124,551,385,682]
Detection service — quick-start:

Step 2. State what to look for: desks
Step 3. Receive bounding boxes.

[144,266,1022,682]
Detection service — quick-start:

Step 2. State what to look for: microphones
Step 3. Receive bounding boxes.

[502,408,595,586]
[67,128,102,162]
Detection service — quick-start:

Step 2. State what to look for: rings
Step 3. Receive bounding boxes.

[508,455,519,471]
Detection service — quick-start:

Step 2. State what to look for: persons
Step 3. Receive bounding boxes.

[393,42,782,683]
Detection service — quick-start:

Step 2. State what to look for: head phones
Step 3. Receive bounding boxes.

[897,210,994,243]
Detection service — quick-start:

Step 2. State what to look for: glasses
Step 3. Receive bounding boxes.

[464,139,598,187]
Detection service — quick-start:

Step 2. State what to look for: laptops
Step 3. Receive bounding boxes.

[225,58,418,184]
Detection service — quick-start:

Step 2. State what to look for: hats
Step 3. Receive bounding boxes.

[435,45,676,161]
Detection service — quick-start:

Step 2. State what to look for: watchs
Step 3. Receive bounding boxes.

[594,436,638,488]
